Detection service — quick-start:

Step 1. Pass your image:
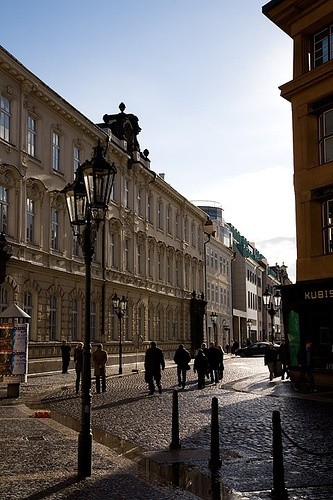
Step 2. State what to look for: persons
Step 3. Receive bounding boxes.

[292,341,318,392]
[60,340,72,374]
[144,341,165,395]
[93,344,108,393]
[225,339,290,381]
[73,342,84,393]
[174,344,190,389]
[193,341,223,389]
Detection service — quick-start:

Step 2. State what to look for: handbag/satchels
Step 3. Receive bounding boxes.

[144,370,150,383]
[216,366,223,380]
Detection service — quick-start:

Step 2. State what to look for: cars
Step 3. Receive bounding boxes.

[235,342,280,357]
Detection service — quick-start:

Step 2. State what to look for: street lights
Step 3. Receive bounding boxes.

[61,137,117,476]
[263,286,281,350]
[111,292,128,374]
[210,312,218,347]
[247,319,252,340]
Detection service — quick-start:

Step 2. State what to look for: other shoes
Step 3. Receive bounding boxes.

[178,383,186,388]
[198,386,206,389]
[215,380,220,383]
[148,391,154,395]
[62,372,69,375]
[211,378,214,382]
[76,389,78,394]
[159,388,163,394]
